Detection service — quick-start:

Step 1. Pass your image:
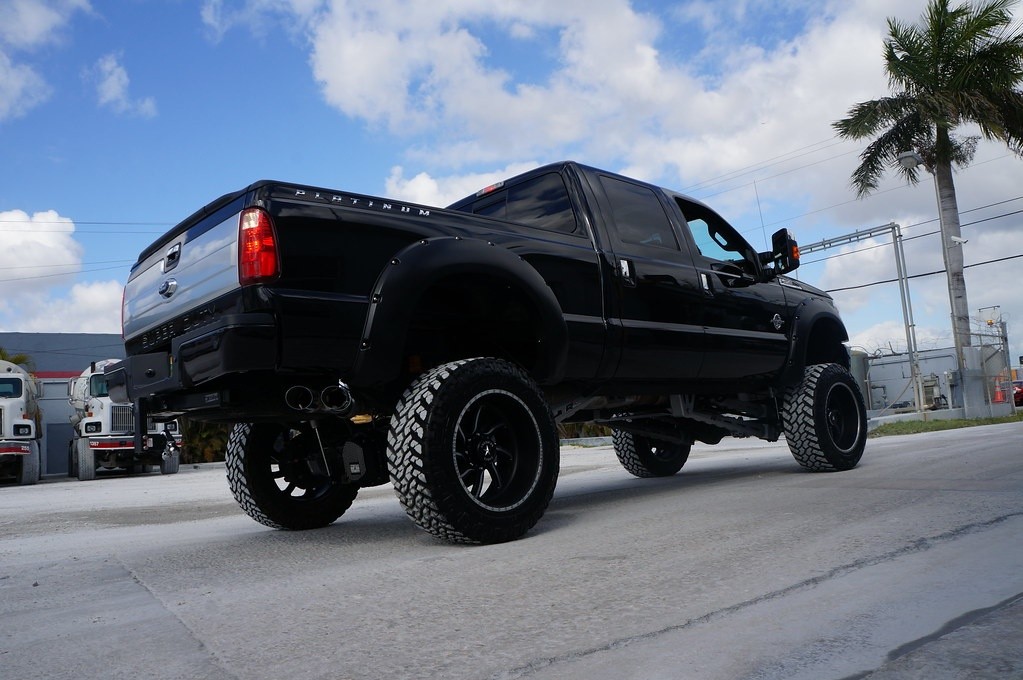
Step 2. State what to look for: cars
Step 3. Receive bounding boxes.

[997,380,1023,407]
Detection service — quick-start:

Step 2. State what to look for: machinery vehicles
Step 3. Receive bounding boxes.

[0,360,42,483]
[69,358,182,481]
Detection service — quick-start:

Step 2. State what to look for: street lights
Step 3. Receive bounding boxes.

[897,152,969,419]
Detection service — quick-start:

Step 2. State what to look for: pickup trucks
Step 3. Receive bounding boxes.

[119,158,870,542]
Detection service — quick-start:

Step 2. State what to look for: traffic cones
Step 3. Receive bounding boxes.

[992,376,1006,404]
